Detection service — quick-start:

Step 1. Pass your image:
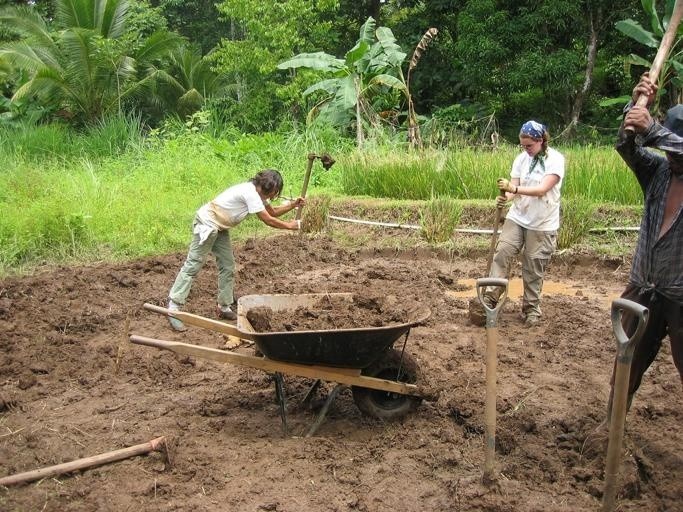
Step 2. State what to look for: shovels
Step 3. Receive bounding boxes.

[469,188,506,326]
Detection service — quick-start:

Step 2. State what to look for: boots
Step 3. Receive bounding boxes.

[595,396,631,434]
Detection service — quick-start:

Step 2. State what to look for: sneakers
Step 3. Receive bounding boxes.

[167,315,188,333]
[216,306,236,320]
[525,315,538,325]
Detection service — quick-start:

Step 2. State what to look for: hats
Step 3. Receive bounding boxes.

[663,104,682,135]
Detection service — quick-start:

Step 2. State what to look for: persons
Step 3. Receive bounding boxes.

[483,120,566,323]
[165,169,307,332]
[594,69,683,439]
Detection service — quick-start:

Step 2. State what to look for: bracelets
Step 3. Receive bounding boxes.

[513,186,518,194]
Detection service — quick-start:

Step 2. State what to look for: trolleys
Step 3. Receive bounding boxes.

[130,292,437,416]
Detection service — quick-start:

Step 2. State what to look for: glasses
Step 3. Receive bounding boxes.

[519,143,537,149]
